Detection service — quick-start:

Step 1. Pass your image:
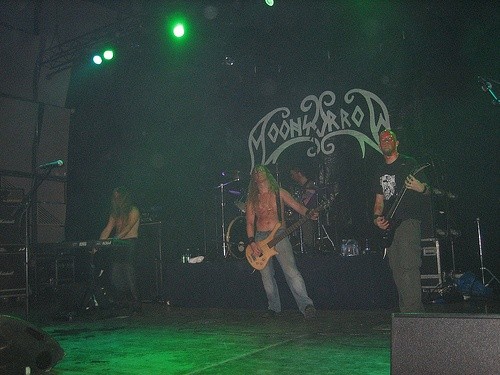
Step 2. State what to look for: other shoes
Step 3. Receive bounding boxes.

[260,310,276,320]
[305,304,317,321]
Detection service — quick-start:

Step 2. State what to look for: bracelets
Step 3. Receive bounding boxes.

[248,237,255,243]
[305,209,311,215]
[374,215,380,219]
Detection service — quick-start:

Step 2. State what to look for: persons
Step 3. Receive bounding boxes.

[290,166,316,245]
[372,129,432,312]
[244,163,319,322]
[91,186,144,316]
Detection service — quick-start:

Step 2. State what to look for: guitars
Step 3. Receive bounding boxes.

[373,168,416,249]
[245,204,326,271]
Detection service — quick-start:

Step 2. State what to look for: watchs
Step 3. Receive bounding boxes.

[422,185,430,193]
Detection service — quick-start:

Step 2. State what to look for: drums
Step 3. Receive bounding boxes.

[225,215,247,260]
[281,182,304,202]
[221,170,242,194]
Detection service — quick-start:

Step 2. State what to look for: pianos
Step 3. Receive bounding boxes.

[53,238,138,253]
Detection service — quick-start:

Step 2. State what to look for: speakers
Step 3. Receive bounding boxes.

[0,199,28,249]
[0,251,28,294]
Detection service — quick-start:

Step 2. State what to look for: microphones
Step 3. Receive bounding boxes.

[40,160,65,170]
